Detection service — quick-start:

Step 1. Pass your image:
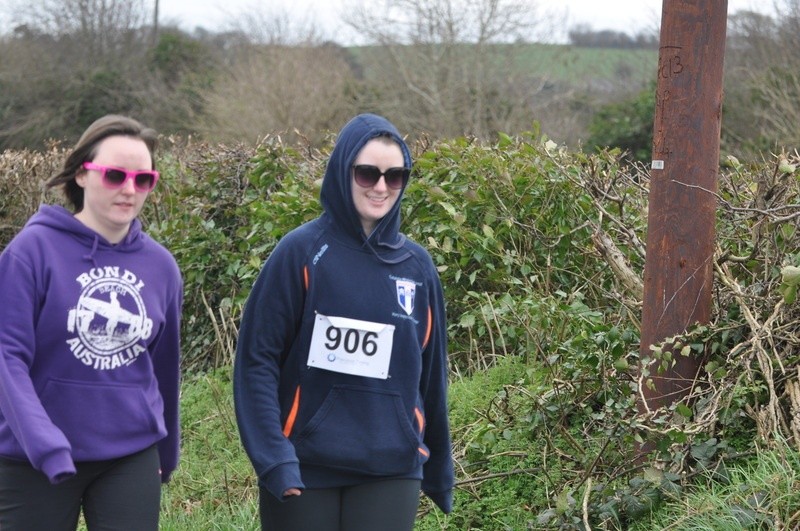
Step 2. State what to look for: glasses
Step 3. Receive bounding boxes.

[350,164,411,190]
[81,161,161,193]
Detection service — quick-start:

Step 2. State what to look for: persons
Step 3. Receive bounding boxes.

[1,115,188,531]
[232,112,455,531]
